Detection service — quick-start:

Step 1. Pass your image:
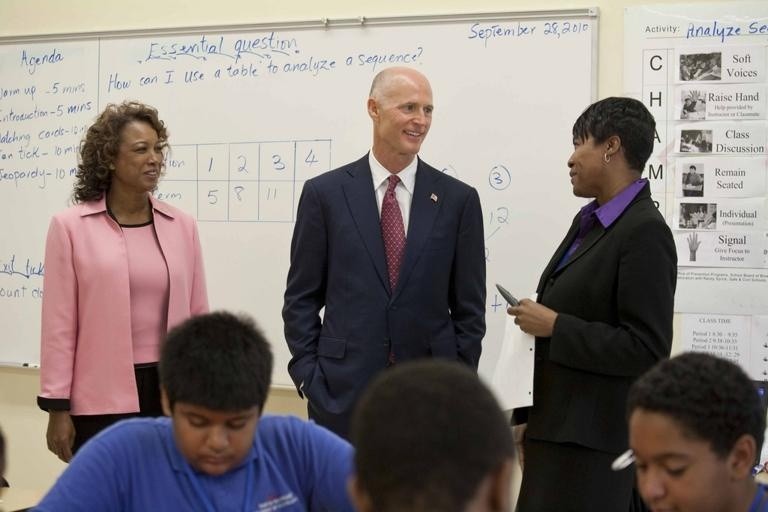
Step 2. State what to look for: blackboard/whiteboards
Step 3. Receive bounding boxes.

[0,6,599,411]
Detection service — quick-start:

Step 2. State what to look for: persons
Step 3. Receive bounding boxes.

[27,307,358,512]
[278,65,489,443]
[36,99,211,463]
[508,95,679,511]
[685,165,703,185]
[344,358,518,511]
[683,98,697,112]
[624,349,768,512]
[686,232,701,261]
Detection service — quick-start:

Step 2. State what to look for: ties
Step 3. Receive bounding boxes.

[382,176,405,293]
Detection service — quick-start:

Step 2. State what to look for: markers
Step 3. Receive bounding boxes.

[23,363,40,368]
